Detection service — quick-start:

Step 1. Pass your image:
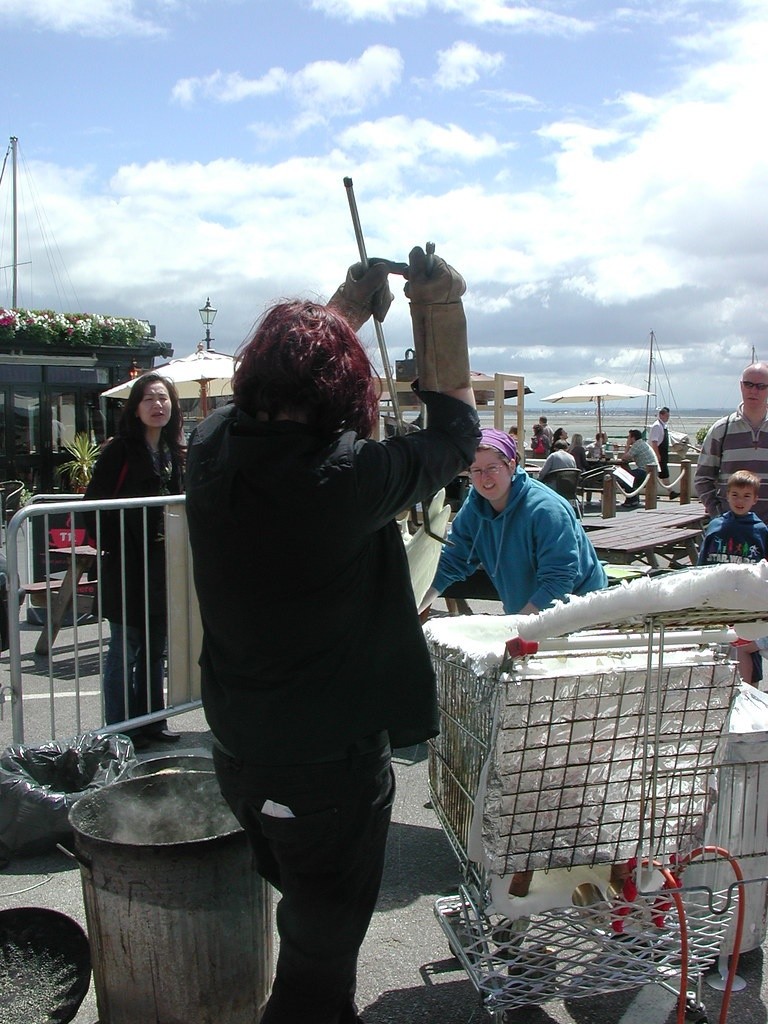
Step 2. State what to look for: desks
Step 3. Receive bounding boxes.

[34,545,111,656]
[586,500,709,577]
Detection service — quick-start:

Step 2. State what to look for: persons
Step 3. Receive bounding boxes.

[621,430,662,507]
[416,428,610,615]
[82,375,190,749]
[649,407,680,501]
[185,241,483,1024]
[510,415,608,507]
[694,362,768,527]
[696,470,768,567]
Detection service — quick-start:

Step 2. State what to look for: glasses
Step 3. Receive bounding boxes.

[467,463,506,479]
[743,381,768,390]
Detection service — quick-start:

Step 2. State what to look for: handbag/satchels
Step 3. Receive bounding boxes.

[533,435,545,454]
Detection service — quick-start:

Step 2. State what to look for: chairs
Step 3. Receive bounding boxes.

[541,462,625,520]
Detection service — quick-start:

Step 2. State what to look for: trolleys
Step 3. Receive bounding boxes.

[420,557,768,1024]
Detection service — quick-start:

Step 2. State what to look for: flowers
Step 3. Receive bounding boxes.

[0,308,152,348]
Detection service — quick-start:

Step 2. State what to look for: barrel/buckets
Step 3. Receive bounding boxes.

[55,770,273,1024]
[125,754,215,778]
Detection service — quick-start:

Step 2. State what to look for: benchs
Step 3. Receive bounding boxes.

[22,577,97,597]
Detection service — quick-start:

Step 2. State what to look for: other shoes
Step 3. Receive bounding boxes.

[669,491,680,499]
[131,733,151,752]
[622,500,639,508]
[147,726,180,742]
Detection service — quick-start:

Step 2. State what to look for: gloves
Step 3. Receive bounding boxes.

[325,257,408,333]
[403,246,470,393]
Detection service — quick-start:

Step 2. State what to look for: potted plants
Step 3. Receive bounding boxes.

[55,432,99,492]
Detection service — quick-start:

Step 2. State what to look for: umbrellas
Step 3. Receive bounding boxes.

[539,376,657,459]
[471,371,535,404]
[101,341,241,419]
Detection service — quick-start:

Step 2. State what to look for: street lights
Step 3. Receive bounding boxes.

[198,295,217,350]
